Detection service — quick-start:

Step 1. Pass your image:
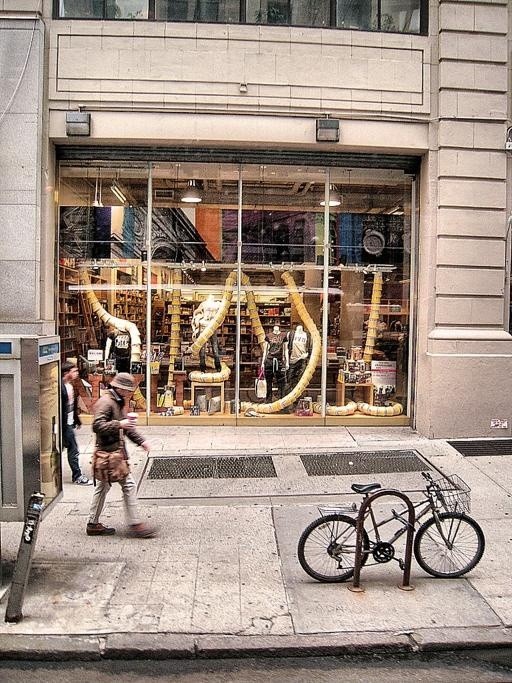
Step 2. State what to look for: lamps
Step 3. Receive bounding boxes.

[314,116,341,144]
[319,189,341,209]
[64,109,92,136]
[180,183,202,205]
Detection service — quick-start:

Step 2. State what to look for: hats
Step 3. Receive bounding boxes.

[110,373,134,392]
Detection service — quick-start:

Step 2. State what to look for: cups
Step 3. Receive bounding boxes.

[126,410,138,428]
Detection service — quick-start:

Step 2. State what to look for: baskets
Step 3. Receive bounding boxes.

[434,475,472,516]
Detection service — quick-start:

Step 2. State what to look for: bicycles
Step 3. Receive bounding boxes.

[245,362,309,405]
[296,472,486,584]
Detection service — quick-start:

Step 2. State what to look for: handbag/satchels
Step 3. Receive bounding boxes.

[92,450,130,483]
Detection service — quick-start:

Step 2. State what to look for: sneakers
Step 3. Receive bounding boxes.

[74,475,93,486]
[87,523,114,536]
[129,526,158,538]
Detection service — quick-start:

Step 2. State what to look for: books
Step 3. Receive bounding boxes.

[180,302,292,360]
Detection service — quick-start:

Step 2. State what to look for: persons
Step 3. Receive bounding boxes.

[60,358,92,486]
[104,325,133,372]
[86,372,159,539]
[261,326,289,413]
[194,293,222,373]
[285,325,310,412]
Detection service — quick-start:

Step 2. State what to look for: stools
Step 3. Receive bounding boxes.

[191,381,226,414]
[334,380,373,410]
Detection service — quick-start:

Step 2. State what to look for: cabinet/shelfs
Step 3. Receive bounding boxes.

[56,259,409,378]
